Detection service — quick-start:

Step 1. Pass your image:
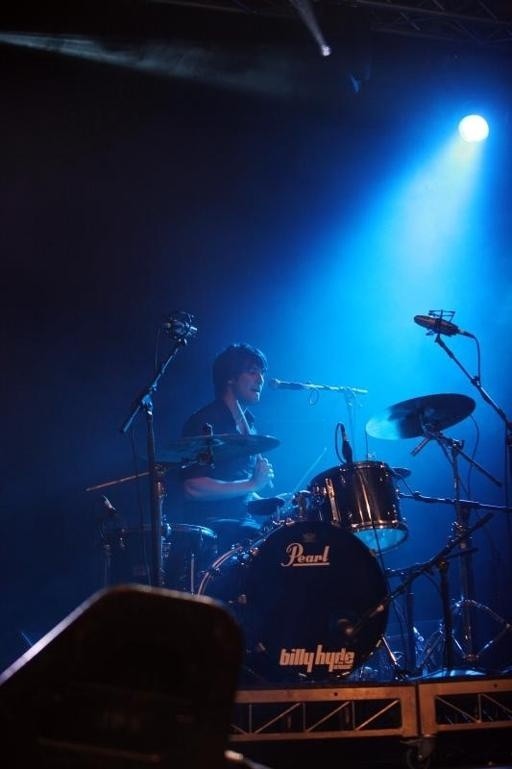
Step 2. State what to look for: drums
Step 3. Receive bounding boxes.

[307,460,411,554]
[199,520,392,688]
[159,522,214,587]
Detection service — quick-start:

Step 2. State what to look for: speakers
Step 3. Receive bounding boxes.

[0,583,246,767]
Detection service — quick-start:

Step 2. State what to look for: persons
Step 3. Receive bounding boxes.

[181,343,274,554]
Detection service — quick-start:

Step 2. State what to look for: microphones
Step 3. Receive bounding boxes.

[168,319,200,340]
[267,378,304,391]
[414,314,458,337]
[341,425,352,464]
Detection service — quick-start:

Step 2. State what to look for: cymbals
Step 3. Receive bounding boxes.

[365,392,477,441]
[140,432,281,466]
[390,466,412,484]
